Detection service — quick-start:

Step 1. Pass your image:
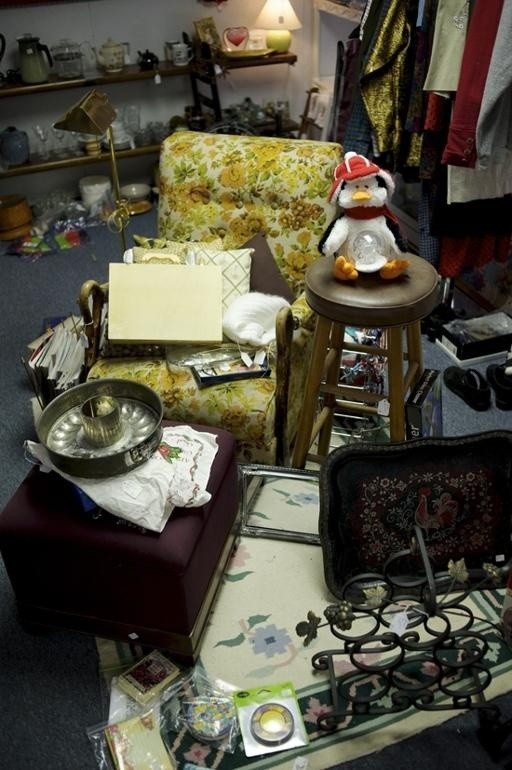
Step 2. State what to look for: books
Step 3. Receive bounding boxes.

[117,650,181,705]
[26,314,89,412]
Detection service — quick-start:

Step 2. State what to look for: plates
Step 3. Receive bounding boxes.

[224,49,275,57]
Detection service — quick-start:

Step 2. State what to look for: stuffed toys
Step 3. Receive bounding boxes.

[317,151,414,283]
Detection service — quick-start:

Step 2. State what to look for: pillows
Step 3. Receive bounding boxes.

[238,233,296,303]
[185,246,255,320]
[130,232,223,268]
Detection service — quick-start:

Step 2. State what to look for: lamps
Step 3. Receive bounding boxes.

[251,1,303,53]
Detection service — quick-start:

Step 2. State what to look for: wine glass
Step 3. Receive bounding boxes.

[70,131,79,150]
[33,125,52,160]
[51,123,67,155]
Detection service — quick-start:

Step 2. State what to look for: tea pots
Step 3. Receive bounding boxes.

[137,49,159,70]
[92,36,131,72]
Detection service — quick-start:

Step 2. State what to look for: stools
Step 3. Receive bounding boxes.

[290,248,440,470]
[1,417,243,664]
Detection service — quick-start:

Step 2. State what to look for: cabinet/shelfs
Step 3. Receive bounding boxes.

[312,0,420,258]
[0,48,301,239]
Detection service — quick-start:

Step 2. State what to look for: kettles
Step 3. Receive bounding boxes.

[15,33,53,82]
[50,39,94,77]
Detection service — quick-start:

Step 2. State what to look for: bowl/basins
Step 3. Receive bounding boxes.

[120,183,151,202]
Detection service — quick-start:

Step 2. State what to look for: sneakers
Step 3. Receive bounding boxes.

[486,363,512,412]
[444,365,491,411]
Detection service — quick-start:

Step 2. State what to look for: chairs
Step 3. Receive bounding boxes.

[79,131,347,466]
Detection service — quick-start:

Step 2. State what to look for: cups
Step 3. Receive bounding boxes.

[164,40,194,66]
[109,103,165,145]
